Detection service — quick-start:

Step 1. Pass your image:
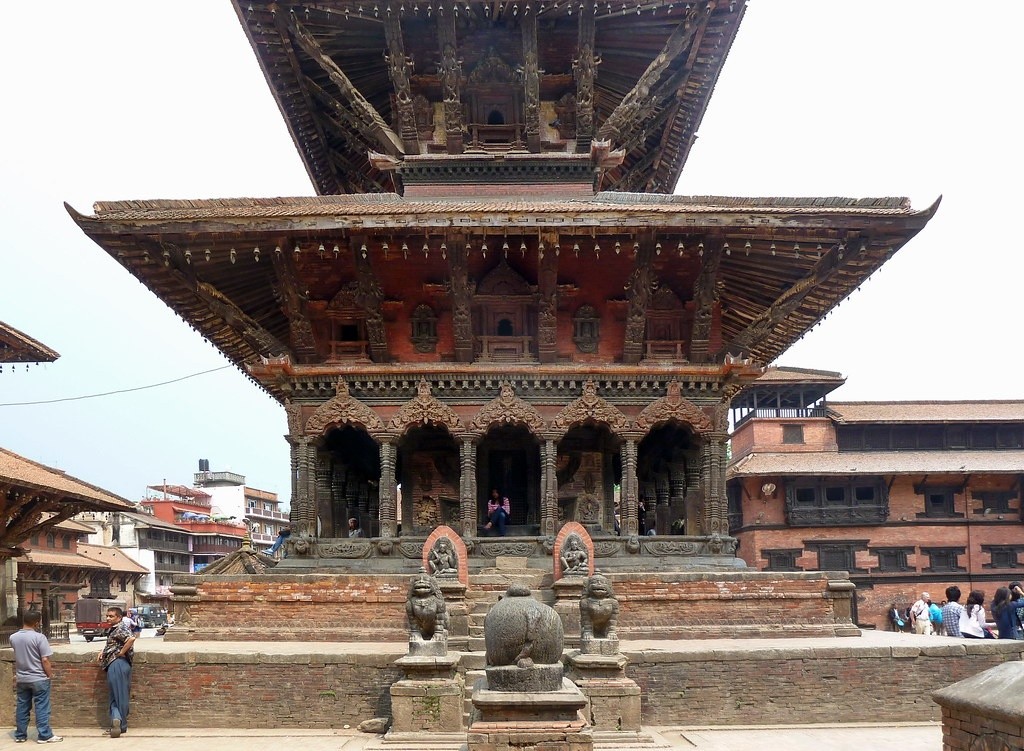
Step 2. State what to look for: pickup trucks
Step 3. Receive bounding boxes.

[76,606,166,642]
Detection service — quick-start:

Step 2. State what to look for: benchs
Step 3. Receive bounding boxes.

[476,524,541,536]
[892,603,992,632]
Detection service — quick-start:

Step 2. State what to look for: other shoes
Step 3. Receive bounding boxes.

[479,527,489,537]
[110,718,122,738]
[261,549,273,556]
[13,734,26,742]
[36,735,63,744]
[101,726,127,736]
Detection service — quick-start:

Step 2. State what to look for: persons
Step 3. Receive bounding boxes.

[910,592,930,635]
[928,600,946,636]
[990,586,1024,640]
[614,502,620,537]
[429,543,455,574]
[639,501,647,535]
[959,590,995,639]
[905,603,916,623]
[123,611,137,627]
[97,607,136,738]
[482,485,510,537]
[261,512,291,560]
[348,517,365,537]
[942,585,965,638]
[561,539,588,571]
[10,612,63,744]
[1009,582,1024,640]
[889,603,904,632]
